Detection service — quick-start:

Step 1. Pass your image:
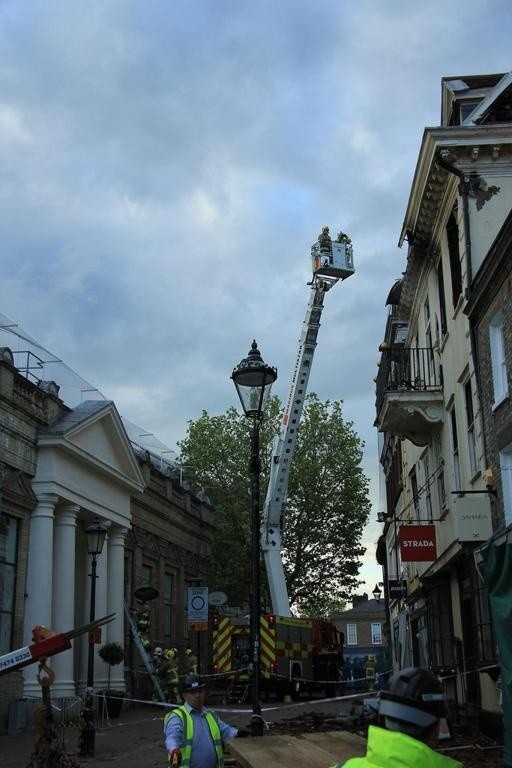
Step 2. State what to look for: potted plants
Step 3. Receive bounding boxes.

[98,641,124,720]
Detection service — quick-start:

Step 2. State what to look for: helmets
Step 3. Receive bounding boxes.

[152,646,192,658]
[375,667,449,727]
[368,655,375,661]
[178,674,206,692]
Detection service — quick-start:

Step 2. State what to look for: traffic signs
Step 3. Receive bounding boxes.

[80,516,109,758]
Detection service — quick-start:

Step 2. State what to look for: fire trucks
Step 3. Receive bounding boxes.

[210,241,354,705]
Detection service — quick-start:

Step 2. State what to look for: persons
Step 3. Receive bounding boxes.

[163,675,248,768]
[147,643,200,705]
[325,665,465,768]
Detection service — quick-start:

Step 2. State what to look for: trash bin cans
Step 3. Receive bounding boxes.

[8,699,27,737]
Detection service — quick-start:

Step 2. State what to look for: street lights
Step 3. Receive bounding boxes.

[230,338,278,738]
